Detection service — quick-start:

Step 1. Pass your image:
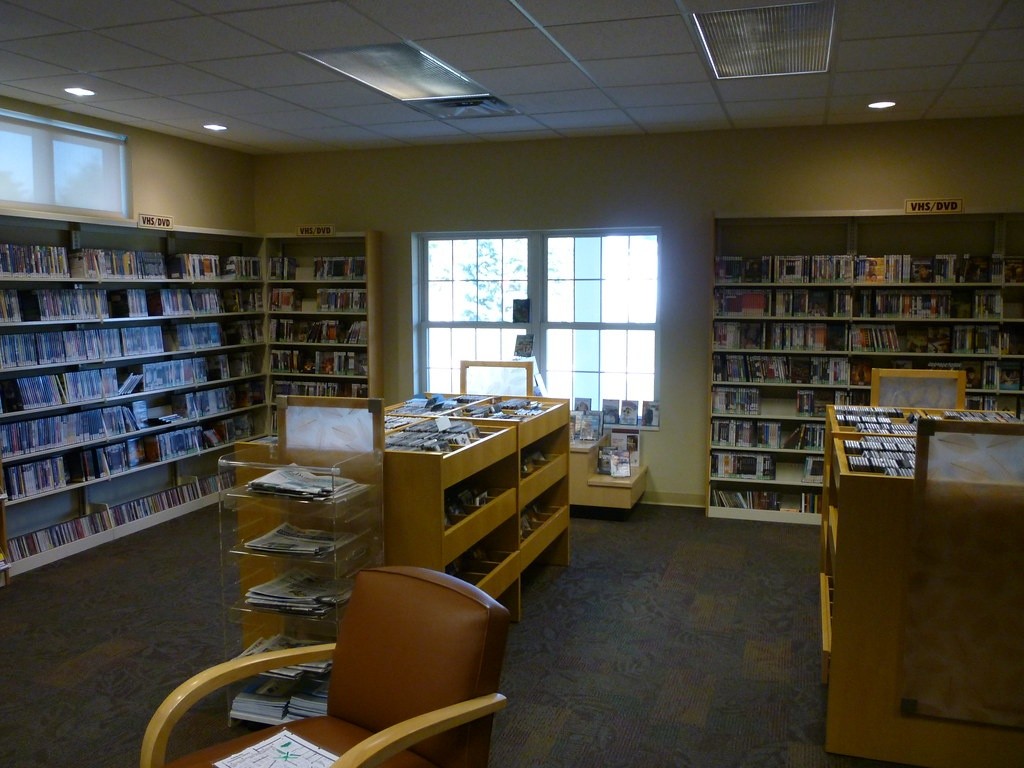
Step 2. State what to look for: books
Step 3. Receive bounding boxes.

[230,676,328,720]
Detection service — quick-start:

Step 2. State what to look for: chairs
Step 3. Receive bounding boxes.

[138,565,509,768]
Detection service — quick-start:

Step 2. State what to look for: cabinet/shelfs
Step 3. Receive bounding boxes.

[231,359,571,655]
[571,432,649,510]
[820,415,1024,767]
[705,197,1024,526]
[0,207,265,590]
[822,367,1023,580]
[216,395,387,729]
[266,226,385,438]
